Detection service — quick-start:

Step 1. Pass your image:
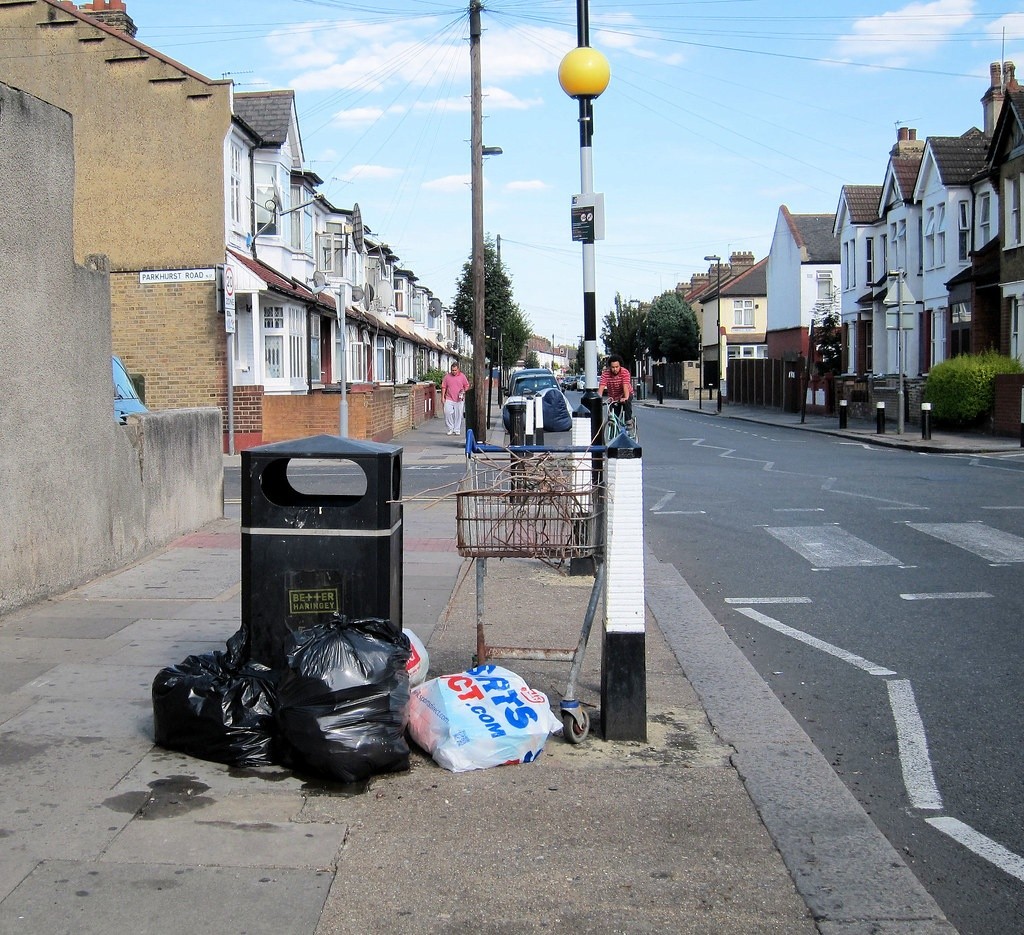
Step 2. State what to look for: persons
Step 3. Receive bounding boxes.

[441,362,469,435]
[597,354,634,439]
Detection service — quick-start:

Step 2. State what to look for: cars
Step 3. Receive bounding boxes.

[561,375,580,391]
[509,368,554,394]
[576,375,586,391]
[582,376,608,396]
[504,372,567,399]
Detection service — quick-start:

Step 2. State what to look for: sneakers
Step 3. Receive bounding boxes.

[447,429,454,435]
[455,431,460,435]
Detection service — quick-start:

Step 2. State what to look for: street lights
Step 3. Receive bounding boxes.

[577,335,583,360]
[499,331,506,383]
[524,344,530,367]
[556,44,612,412]
[702,254,725,413]
[629,299,646,399]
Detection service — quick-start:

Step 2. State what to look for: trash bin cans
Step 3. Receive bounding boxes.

[239,434,404,633]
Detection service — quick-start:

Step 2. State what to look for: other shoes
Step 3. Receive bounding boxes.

[626,419,634,430]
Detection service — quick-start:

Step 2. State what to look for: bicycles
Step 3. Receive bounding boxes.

[602,397,639,448]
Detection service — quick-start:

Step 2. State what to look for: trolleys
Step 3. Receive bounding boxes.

[454,429,612,745]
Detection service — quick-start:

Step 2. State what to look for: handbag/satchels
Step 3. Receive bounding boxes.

[403,628,430,685]
[406,665,564,773]
